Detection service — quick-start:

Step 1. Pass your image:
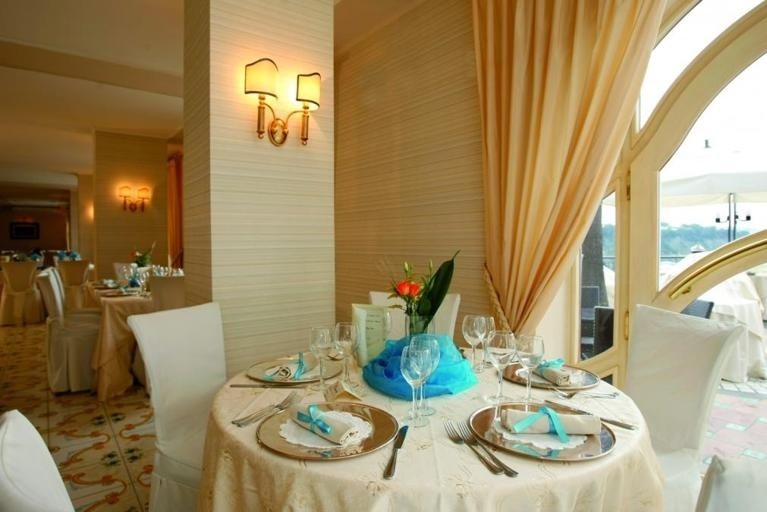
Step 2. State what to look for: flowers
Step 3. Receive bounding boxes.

[387,249,461,336]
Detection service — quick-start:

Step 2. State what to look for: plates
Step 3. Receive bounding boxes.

[257,402,397,462]
[90,280,127,298]
[469,401,615,465]
[502,365,601,389]
[249,360,342,382]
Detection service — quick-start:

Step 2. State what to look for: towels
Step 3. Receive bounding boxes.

[532,365,570,385]
[500,409,601,433]
[279,353,318,378]
[290,406,358,444]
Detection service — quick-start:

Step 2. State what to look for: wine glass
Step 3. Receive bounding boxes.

[309,319,362,393]
[399,334,441,429]
[463,316,495,369]
[516,334,543,404]
[489,331,515,403]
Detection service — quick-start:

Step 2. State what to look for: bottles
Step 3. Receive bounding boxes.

[116,263,185,295]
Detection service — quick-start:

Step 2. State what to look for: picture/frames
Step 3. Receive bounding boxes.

[10,222,40,239]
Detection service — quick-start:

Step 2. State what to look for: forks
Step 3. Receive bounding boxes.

[232,391,302,428]
[457,419,519,477]
[442,420,502,477]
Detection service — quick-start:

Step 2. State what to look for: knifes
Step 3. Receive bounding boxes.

[382,424,408,480]
[530,395,635,428]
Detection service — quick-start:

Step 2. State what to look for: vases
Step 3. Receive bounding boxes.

[406,315,434,337]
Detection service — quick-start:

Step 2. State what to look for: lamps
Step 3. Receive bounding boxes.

[118,186,150,212]
[245,58,321,146]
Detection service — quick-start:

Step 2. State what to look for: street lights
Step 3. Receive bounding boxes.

[712,192,752,245]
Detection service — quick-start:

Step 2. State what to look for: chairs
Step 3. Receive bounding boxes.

[127,302,226,512]
[369,291,460,340]
[581,287,714,384]
[622,303,747,512]
[0,409,75,512]
[0,258,185,403]
[695,455,767,512]
